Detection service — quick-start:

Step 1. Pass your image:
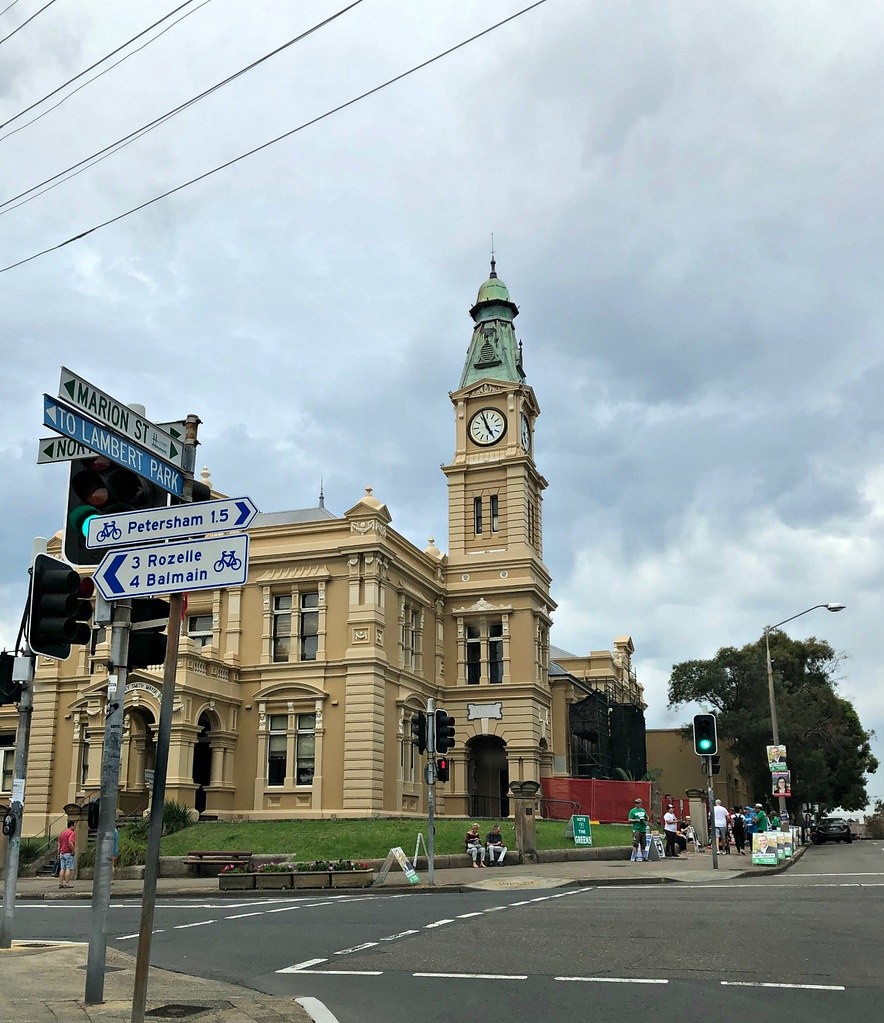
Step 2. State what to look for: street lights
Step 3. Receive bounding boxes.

[764,601,846,833]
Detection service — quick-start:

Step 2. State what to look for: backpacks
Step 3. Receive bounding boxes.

[733,813,743,832]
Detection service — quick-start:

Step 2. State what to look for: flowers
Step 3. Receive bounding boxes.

[222,861,369,873]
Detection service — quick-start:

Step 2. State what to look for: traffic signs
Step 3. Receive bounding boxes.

[38,366,262,600]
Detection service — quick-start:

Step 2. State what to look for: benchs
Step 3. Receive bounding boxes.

[183,850,252,874]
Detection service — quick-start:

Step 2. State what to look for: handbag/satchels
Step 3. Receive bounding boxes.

[467,838,482,848]
[53,856,61,874]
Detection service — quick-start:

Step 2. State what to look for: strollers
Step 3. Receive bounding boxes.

[719,831,731,854]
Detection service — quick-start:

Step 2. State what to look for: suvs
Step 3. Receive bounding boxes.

[815,817,852,844]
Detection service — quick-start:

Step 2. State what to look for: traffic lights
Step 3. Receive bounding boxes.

[411,710,426,755]
[65,449,168,565]
[438,759,449,782]
[29,552,95,661]
[127,596,171,673]
[434,709,455,754]
[692,713,718,756]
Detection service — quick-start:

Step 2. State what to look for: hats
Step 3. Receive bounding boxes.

[684,815,692,820]
[755,803,763,808]
[667,803,675,811]
[635,798,643,804]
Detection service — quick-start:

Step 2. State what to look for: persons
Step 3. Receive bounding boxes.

[774,777,789,793]
[805,813,812,844]
[727,802,780,856]
[110,827,118,884]
[486,825,507,867]
[770,748,786,762]
[465,822,487,868]
[57,821,76,890]
[707,798,731,856]
[680,816,701,848]
[663,803,680,858]
[628,797,650,861]
[756,835,776,853]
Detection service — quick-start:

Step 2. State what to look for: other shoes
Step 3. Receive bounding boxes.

[635,856,638,862]
[665,851,672,857]
[736,852,740,855]
[717,852,720,854]
[111,880,115,885]
[59,884,64,889]
[680,850,691,854]
[671,853,680,857]
[479,864,487,867]
[496,861,505,867]
[473,864,479,868]
[642,855,646,861]
[696,841,701,846]
[488,861,494,867]
[741,851,745,855]
[723,850,726,855]
[63,884,74,888]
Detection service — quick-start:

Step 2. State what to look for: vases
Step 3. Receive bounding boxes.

[292,870,333,890]
[217,873,256,891]
[331,868,375,888]
[254,873,295,889]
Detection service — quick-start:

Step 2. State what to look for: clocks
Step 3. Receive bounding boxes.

[467,406,508,447]
[521,413,531,452]
